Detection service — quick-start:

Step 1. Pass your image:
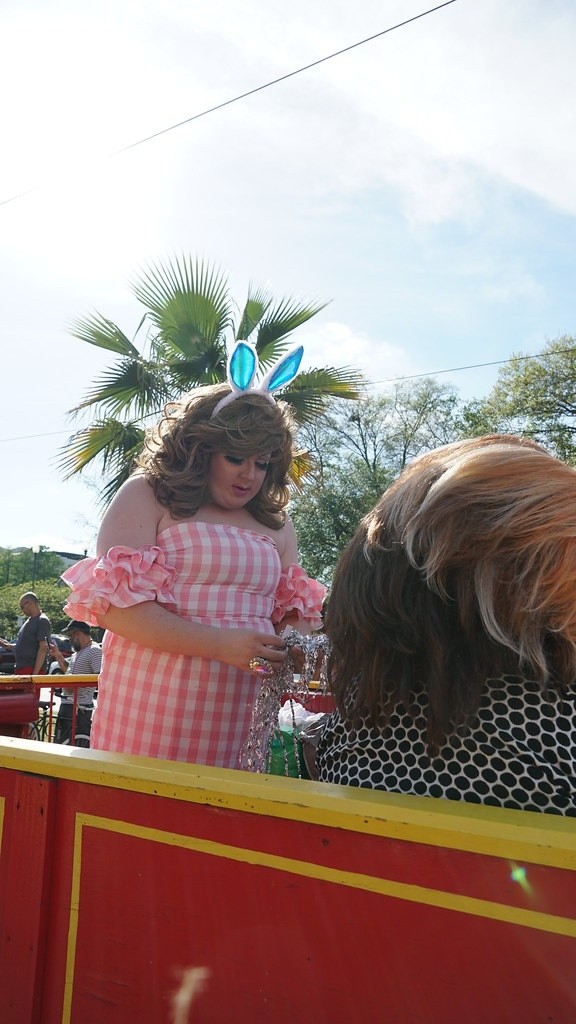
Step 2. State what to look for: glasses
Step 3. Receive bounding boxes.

[20,601,28,610]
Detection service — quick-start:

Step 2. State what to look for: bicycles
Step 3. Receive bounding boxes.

[14,700,90,748]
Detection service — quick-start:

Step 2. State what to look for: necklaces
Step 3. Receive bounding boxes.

[237,627,328,774]
[71,647,84,674]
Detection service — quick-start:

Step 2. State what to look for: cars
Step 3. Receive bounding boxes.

[0,634,103,697]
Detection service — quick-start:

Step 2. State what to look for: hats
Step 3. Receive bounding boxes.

[60,620,90,633]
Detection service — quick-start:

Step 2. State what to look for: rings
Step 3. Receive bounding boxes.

[248,657,274,676]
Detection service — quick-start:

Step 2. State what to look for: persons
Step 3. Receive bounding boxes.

[312,434,576,820]
[44,620,103,748]
[0,592,52,740]
[58,376,329,775]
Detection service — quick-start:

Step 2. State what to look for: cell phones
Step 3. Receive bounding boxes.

[45,637,51,652]
